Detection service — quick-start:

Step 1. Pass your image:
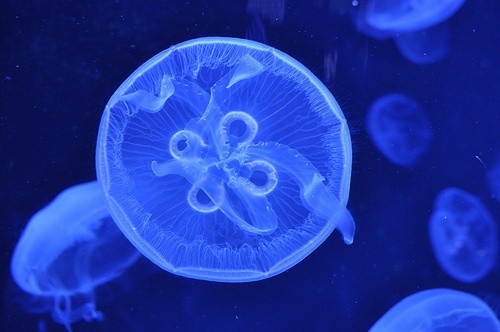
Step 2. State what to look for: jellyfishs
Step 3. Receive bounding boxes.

[364,93,432,168]
[94,36,356,282]
[242,0,285,45]
[11,180,142,332]
[367,287,500,332]
[351,0,467,64]
[427,187,499,284]
